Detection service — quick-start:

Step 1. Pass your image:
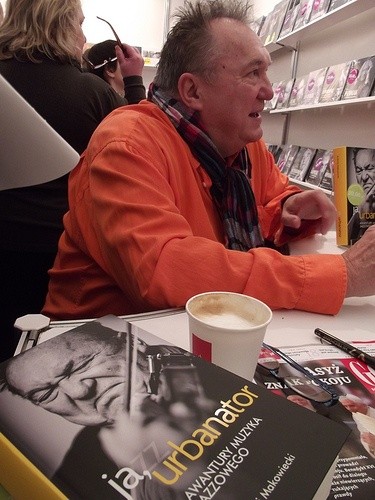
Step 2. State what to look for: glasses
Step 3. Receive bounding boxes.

[258,341,339,408]
[82,16,125,70]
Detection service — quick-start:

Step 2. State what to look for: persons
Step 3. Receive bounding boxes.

[3,320,349,500]
[289,148,312,181]
[87,40,126,101]
[348,148,375,246]
[339,394,375,460]
[43,0,375,315]
[0,1,146,362]
[352,60,372,98]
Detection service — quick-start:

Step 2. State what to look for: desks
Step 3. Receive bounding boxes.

[14,219,375,500]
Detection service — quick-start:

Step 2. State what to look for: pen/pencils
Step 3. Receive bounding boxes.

[315,328,375,369]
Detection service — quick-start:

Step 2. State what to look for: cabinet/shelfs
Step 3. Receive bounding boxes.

[263,0,375,196]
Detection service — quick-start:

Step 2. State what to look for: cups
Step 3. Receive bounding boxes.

[186,291,272,383]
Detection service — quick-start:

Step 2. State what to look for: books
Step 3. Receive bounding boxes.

[330,146,375,248]
[0,315,375,500]
[250,1,375,191]
